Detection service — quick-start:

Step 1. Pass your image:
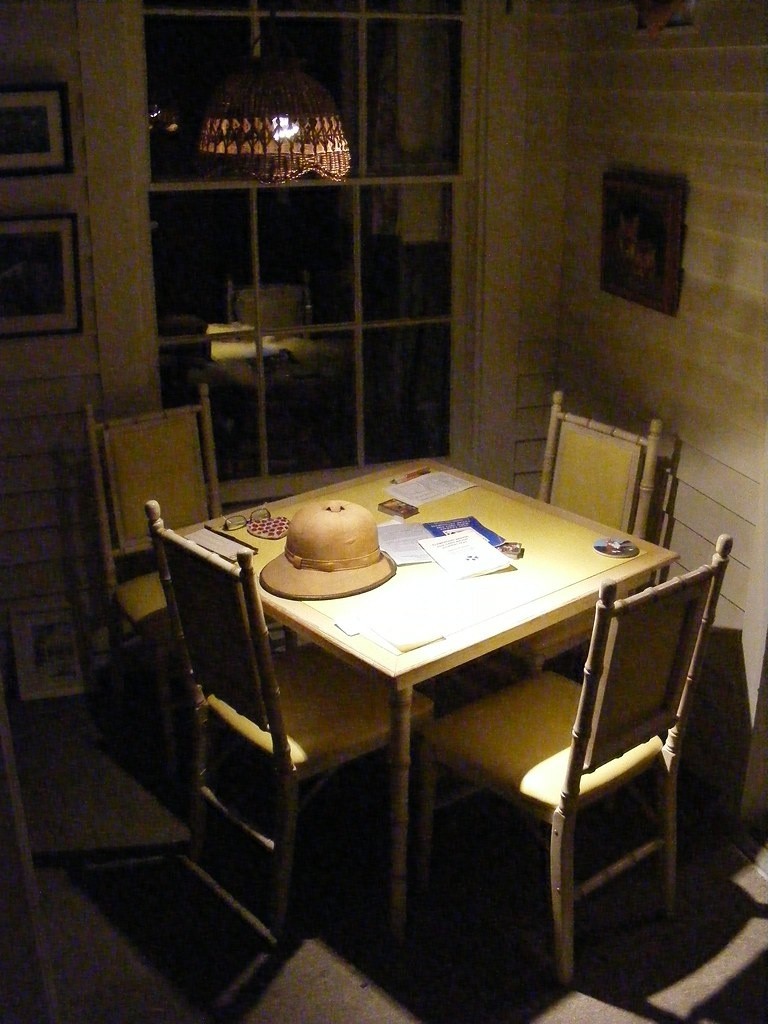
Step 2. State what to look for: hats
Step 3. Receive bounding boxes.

[259,500,397,600]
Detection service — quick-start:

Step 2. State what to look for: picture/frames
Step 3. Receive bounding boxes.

[1,212,85,340]
[602,171,686,319]
[9,596,86,702]
[0,82,78,177]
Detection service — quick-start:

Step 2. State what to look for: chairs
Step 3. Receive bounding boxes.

[424,536,735,984]
[156,268,410,479]
[147,500,437,935]
[83,383,230,760]
[501,391,664,675]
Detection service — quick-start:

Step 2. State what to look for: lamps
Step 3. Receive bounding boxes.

[196,0,352,187]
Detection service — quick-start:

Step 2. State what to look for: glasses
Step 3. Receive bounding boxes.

[219,502,271,531]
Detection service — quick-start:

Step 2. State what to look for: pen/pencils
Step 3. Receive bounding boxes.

[391,466,430,484]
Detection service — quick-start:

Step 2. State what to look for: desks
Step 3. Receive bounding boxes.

[207,323,351,466]
[168,456,679,926]
